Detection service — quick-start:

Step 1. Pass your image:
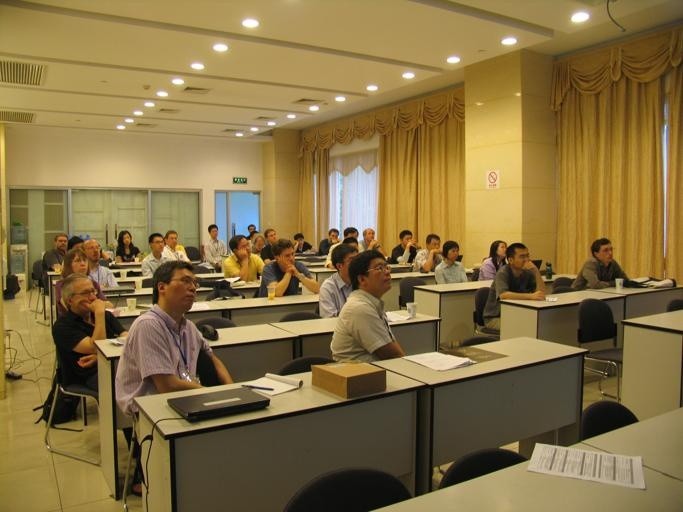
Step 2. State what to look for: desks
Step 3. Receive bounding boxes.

[270,307,442,355]
[378,399,682,512]
[498,280,682,368]
[135,362,427,512]
[372,333,585,494]
[619,305,682,425]
[94,323,298,501]
[29,245,511,334]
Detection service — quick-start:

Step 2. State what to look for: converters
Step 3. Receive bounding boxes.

[5,370,23,380]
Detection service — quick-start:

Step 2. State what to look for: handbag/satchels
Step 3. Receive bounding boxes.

[624,276,676,288]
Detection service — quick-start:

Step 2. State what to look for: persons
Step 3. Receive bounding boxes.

[572,238,629,288]
[483,243,546,334]
[115,261,234,441]
[205,225,311,280]
[52,273,144,494]
[478,241,509,278]
[392,229,469,284]
[319,226,387,318]
[43,231,190,296]
[260,239,320,297]
[330,252,404,364]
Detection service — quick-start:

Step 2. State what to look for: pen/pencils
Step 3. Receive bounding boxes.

[114,295,120,308]
[241,385,274,390]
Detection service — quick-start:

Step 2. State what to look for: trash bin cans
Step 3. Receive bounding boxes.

[3,275,18,300]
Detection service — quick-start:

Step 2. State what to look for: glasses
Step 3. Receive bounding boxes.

[169,277,196,285]
[366,265,391,272]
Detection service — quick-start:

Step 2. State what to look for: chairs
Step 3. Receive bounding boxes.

[575,297,626,405]
[30,258,54,329]
[574,398,642,438]
[469,288,504,341]
[43,335,105,467]
[396,273,431,312]
[280,464,413,512]
[433,441,532,486]
[550,277,581,298]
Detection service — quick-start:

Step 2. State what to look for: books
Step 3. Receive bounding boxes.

[247,373,304,397]
[402,351,476,371]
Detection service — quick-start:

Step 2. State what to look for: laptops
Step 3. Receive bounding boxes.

[167,385,270,422]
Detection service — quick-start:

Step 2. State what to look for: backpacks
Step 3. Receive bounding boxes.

[43,378,80,424]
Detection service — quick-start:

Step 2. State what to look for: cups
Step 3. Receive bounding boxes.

[119,270,126,280]
[614,278,623,289]
[134,279,142,291]
[266,286,275,300]
[125,298,137,313]
[54,264,60,273]
[134,258,139,263]
[405,302,417,319]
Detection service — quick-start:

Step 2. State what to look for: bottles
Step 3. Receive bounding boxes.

[369,239,380,248]
[544,260,551,279]
[109,247,114,261]
